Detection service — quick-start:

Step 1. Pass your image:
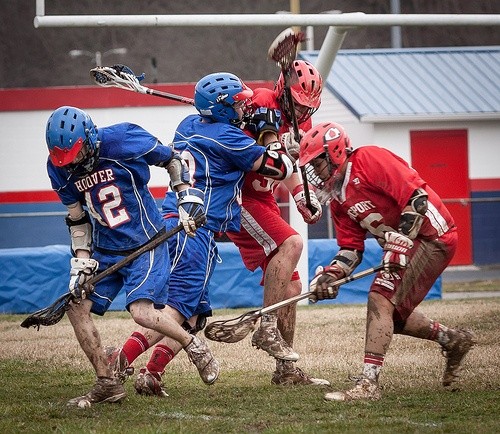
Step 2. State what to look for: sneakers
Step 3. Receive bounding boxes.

[251,325,299,362]
[271,366,330,385]
[68,377,126,407]
[441,327,476,386]
[324,373,381,401]
[186,334,220,385]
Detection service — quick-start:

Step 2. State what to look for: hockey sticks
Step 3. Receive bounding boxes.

[19,214,204,329]
[88,65,200,105]
[204,262,389,343]
[269,26,314,211]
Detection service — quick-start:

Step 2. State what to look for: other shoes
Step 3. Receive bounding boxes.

[113,354,130,385]
[134,373,169,397]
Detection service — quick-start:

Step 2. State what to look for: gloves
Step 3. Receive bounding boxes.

[283,128,305,163]
[175,188,205,237]
[69,257,99,304]
[292,183,323,224]
[308,264,345,303]
[244,87,281,146]
[382,231,414,273]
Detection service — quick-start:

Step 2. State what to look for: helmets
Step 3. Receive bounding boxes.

[45,105,99,177]
[298,121,351,193]
[194,72,253,125]
[273,59,322,127]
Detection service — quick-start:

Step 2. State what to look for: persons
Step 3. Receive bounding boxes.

[46,107,219,408]
[105,72,293,397]
[299,121,476,404]
[226,61,331,387]
